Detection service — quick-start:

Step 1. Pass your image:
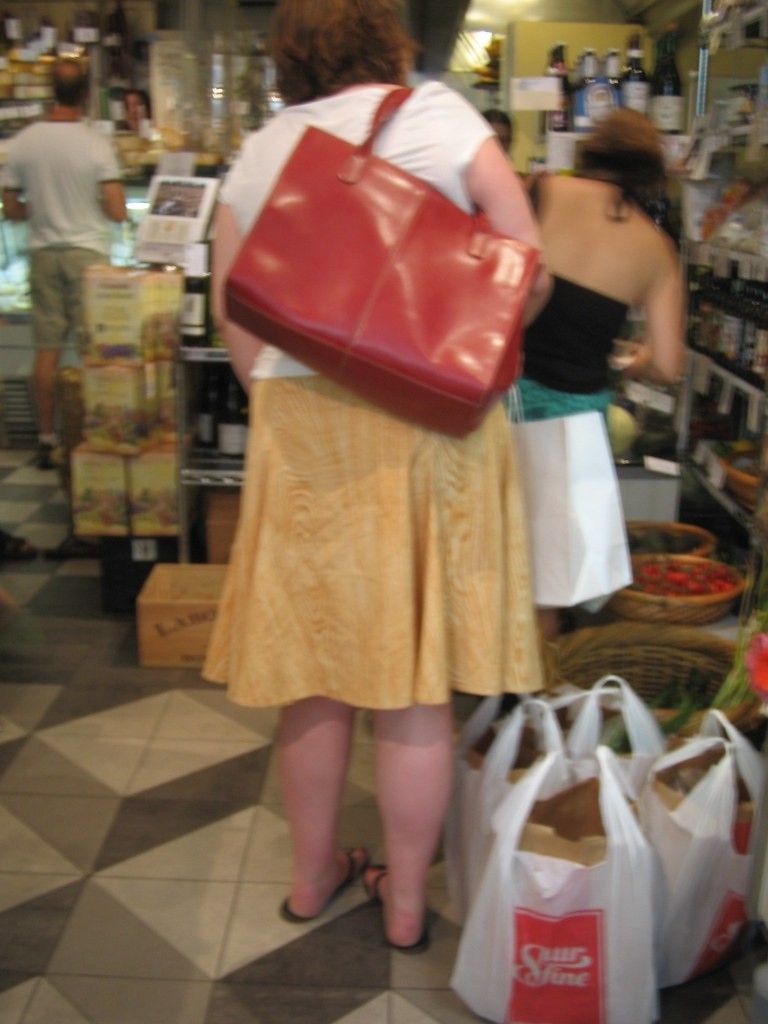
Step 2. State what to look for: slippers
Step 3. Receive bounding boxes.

[363,865,431,954]
[280,845,368,922]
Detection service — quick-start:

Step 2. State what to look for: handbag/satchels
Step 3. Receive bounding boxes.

[502,383,633,608]
[223,89,541,436]
[444,672,767,1024]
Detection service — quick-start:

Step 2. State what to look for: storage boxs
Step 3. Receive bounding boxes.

[70,264,184,537]
[206,491,244,562]
[138,563,233,669]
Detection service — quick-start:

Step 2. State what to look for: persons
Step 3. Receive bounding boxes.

[480,109,513,154]
[511,111,686,646]
[211,1,544,954]
[123,90,150,130]
[0,61,125,469]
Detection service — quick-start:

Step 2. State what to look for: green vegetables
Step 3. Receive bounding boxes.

[641,669,710,736]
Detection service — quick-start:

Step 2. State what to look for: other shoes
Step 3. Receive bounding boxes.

[47,536,94,562]
[0,527,37,562]
[38,438,62,468]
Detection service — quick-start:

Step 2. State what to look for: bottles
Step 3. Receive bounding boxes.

[543,34,685,135]
[193,365,249,459]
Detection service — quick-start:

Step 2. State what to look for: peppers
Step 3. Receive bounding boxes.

[626,559,735,599]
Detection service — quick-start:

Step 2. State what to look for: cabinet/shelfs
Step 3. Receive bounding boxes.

[543,1,767,566]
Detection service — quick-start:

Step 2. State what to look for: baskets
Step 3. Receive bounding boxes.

[624,518,718,560]
[543,621,762,737]
[603,555,744,623]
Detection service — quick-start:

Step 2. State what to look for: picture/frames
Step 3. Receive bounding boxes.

[138,173,221,245]
